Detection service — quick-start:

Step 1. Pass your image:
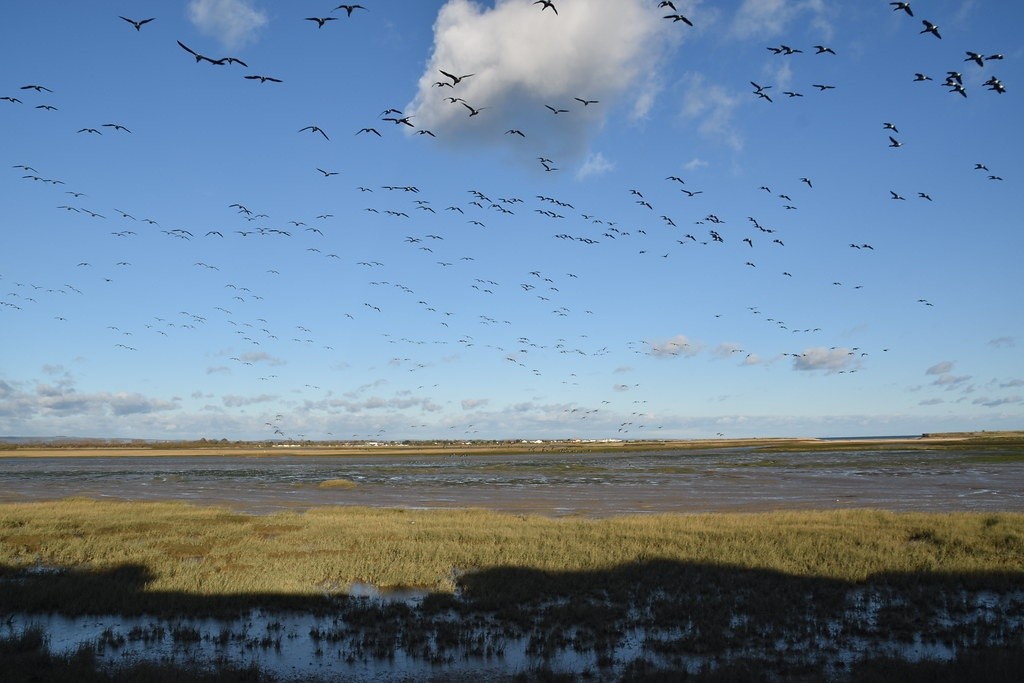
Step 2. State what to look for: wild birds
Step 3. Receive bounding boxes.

[988,176,1003,181]
[920,19,942,39]
[974,163,989,171]
[888,135,903,147]
[883,123,899,133]
[918,300,927,302]
[941,78,958,87]
[985,55,1003,60]
[848,244,861,250]
[862,244,874,250]
[963,52,984,68]
[918,192,932,201]
[890,190,905,201]
[926,303,934,306]
[947,71,963,85]
[949,85,967,98]
[889,2,914,17]
[0,0,888,437]
[912,73,933,81]
[982,75,1006,94]
